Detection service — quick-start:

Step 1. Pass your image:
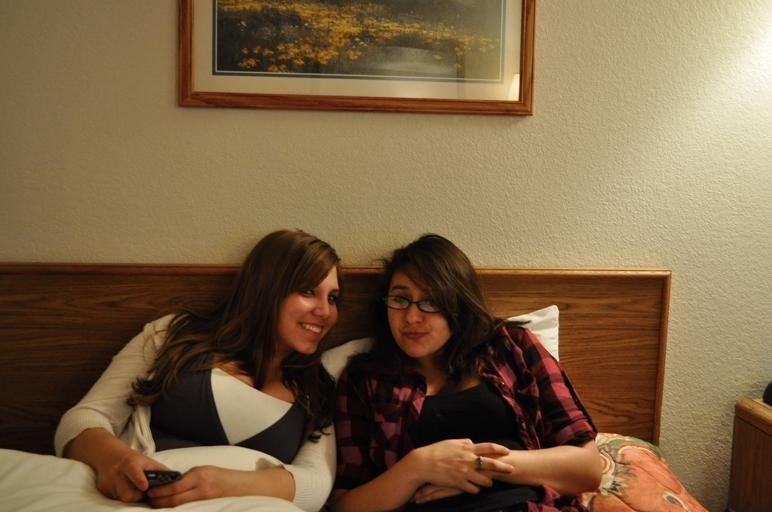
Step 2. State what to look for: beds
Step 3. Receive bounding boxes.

[0,259,715,511]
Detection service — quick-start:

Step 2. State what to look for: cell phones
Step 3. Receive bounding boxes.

[145,468,181,486]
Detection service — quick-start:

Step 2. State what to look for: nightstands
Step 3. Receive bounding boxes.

[727,397,772,511]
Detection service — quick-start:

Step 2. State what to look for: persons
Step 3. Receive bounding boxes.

[51,226,339,512]
[327,232,604,511]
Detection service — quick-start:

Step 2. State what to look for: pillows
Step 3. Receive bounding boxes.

[321,337,376,383]
[507,302,564,365]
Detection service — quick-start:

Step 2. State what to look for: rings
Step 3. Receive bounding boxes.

[110,487,118,500]
[475,452,484,472]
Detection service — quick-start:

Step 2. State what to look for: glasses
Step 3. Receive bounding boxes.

[380,295,441,313]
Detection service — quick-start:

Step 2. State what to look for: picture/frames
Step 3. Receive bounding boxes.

[174,0,540,119]
